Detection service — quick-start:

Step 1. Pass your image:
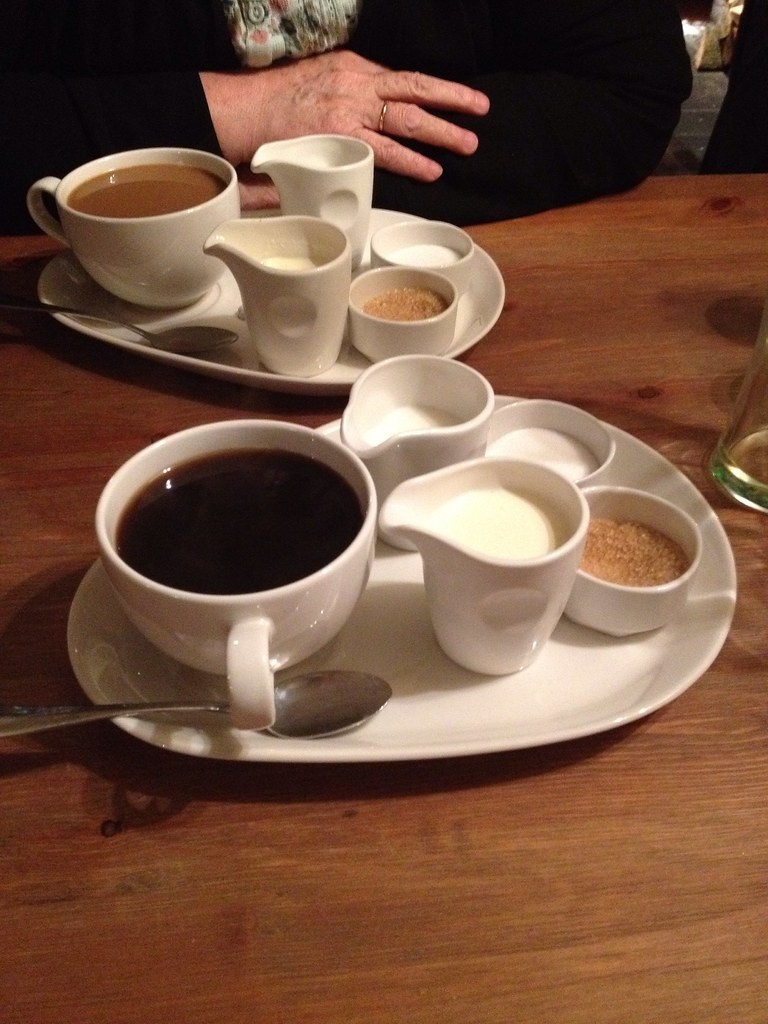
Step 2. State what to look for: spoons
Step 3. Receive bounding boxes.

[0,300,239,352]
[0,670,391,740]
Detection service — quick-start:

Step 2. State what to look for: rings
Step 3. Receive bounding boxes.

[378,100,387,131]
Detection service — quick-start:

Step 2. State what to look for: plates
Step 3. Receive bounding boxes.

[35,209,506,389]
[65,396,738,761]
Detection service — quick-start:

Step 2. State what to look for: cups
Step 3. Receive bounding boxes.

[203,215,351,377]
[379,456,590,675]
[26,147,239,310]
[96,417,378,730]
[251,134,374,271]
[339,355,495,550]
[708,301,768,514]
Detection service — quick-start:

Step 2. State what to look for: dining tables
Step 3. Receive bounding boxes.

[0,171,767,1024]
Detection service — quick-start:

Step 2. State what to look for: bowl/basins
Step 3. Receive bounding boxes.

[371,221,474,299]
[562,487,704,636]
[348,266,458,362]
[485,399,616,486]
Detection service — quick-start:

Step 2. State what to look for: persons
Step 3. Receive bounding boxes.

[0,0,695,238]
